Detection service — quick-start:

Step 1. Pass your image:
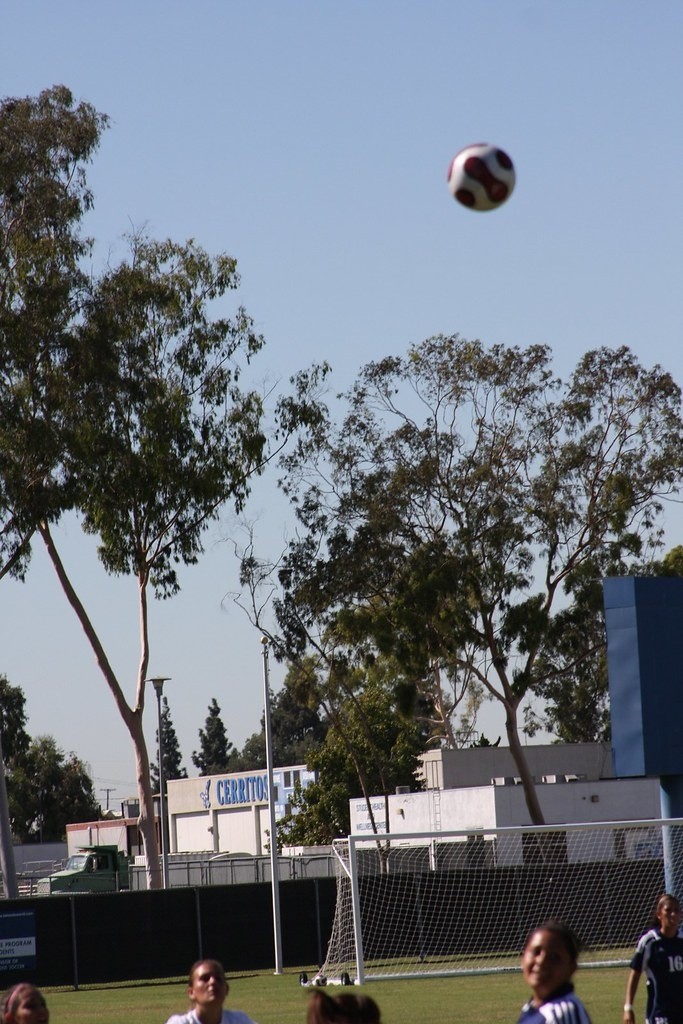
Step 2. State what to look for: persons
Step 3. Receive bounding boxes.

[307,989,380,1024]
[164,960,256,1024]
[0,983,49,1024]
[623,893,683,1024]
[515,920,593,1024]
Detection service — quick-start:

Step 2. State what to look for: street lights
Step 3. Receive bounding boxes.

[143,676,171,881]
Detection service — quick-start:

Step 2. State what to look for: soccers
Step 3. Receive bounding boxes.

[446,142,516,211]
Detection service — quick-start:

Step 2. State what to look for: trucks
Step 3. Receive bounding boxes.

[35,844,138,895]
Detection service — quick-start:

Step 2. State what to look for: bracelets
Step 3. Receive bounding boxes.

[624,1005,632,1011]
